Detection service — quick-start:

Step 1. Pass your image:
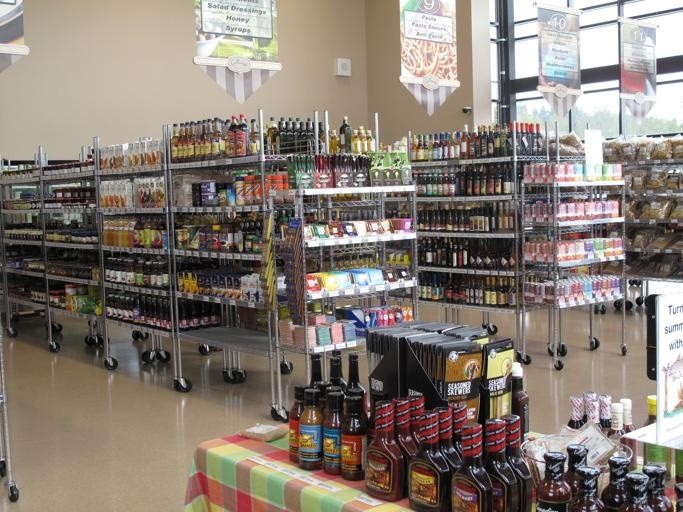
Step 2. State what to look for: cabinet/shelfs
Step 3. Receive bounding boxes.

[0,107,683,422]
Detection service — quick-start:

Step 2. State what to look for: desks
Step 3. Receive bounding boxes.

[184,422,563,512]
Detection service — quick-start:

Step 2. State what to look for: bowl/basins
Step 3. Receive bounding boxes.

[521,430,635,498]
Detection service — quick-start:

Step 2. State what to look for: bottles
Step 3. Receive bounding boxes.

[618,398,636,436]
[503,414,536,512]
[391,398,417,465]
[0,111,548,348]
[598,456,631,512]
[509,359,531,437]
[328,350,348,389]
[673,481,683,512]
[571,465,604,512]
[410,414,449,512]
[534,452,572,512]
[339,388,367,483]
[407,394,424,434]
[609,402,624,437]
[434,406,462,476]
[287,385,302,463]
[480,419,523,511]
[322,385,342,475]
[641,394,657,426]
[586,390,602,431]
[565,444,588,495]
[450,403,468,440]
[366,390,404,502]
[451,427,493,512]
[617,471,654,511]
[304,353,327,391]
[344,349,368,401]
[599,395,613,431]
[299,388,324,470]
[641,464,674,511]
[568,396,584,430]
